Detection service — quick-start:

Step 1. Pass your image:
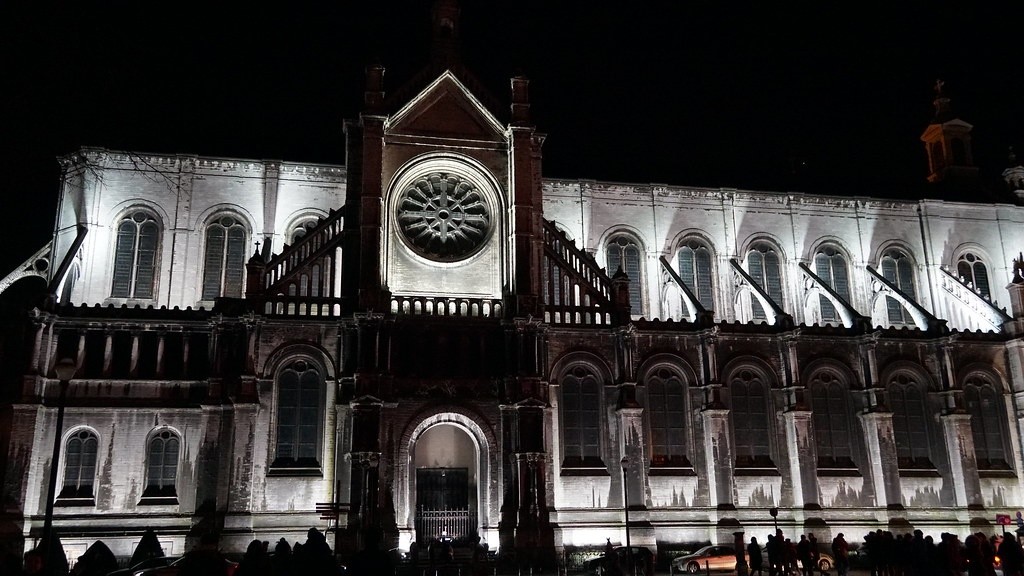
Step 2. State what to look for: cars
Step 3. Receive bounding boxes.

[671,544,745,573]
[582,547,656,575]
[750,543,835,571]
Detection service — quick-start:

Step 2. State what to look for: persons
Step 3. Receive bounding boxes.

[0,528,1024,576]
